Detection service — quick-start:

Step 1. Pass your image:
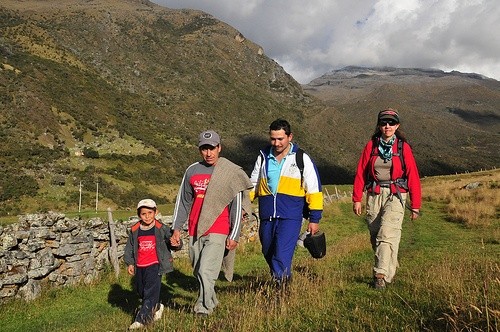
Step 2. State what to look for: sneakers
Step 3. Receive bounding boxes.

[375,278,386,290]
[129,321,144,330]
[153,304,164,321]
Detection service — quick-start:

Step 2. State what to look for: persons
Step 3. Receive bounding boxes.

[170,131,254,321]
[122,198,183,330]
[240,118,323,290]
[352,107,421,285]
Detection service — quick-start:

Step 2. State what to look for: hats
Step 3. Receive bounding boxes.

[378,108,399,123]
[137,199,157,209]
[198,131,221,147]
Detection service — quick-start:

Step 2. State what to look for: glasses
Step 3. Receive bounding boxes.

[379,119,397,126]
[200,146,216,150]
